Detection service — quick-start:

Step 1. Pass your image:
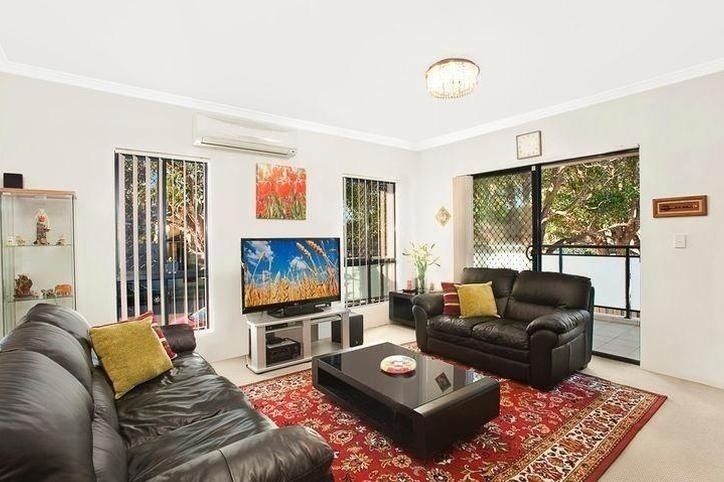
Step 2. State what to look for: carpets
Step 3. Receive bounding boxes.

[240,340,668,482]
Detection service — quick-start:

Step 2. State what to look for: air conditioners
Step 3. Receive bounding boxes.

[191,114,298,158]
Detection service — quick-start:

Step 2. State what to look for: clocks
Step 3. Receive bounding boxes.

[516,130,542,159]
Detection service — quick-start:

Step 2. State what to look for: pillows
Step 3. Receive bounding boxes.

[441,282,460,316]
[92,309,179,360]
[89,315,174,400]
[452,281,502,319]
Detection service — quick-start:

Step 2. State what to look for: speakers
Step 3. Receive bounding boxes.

[3,173,22,189]
[331,312,363,347]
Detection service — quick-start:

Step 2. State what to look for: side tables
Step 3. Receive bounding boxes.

[389,290,440,329]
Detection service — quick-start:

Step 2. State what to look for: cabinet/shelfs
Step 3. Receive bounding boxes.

[0,188,77,340]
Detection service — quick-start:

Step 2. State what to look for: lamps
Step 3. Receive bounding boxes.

[426,58,481,99]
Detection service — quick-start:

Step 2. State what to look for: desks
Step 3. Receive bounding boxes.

[116,263,205,282]
[243,304,351,374]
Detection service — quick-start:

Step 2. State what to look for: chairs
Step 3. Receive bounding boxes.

[116,244,183,293]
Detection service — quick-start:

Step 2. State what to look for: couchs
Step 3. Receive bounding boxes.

[410,267,594,391]
[0,303,336,481]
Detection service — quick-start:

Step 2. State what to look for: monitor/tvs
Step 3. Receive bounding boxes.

[241,238,341,318]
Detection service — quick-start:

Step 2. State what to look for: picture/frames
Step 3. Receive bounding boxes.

[652,195,707,218]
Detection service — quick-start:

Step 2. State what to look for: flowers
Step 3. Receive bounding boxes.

[402,240,441,267]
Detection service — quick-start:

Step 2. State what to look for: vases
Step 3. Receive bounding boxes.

[414,260,427,295]
